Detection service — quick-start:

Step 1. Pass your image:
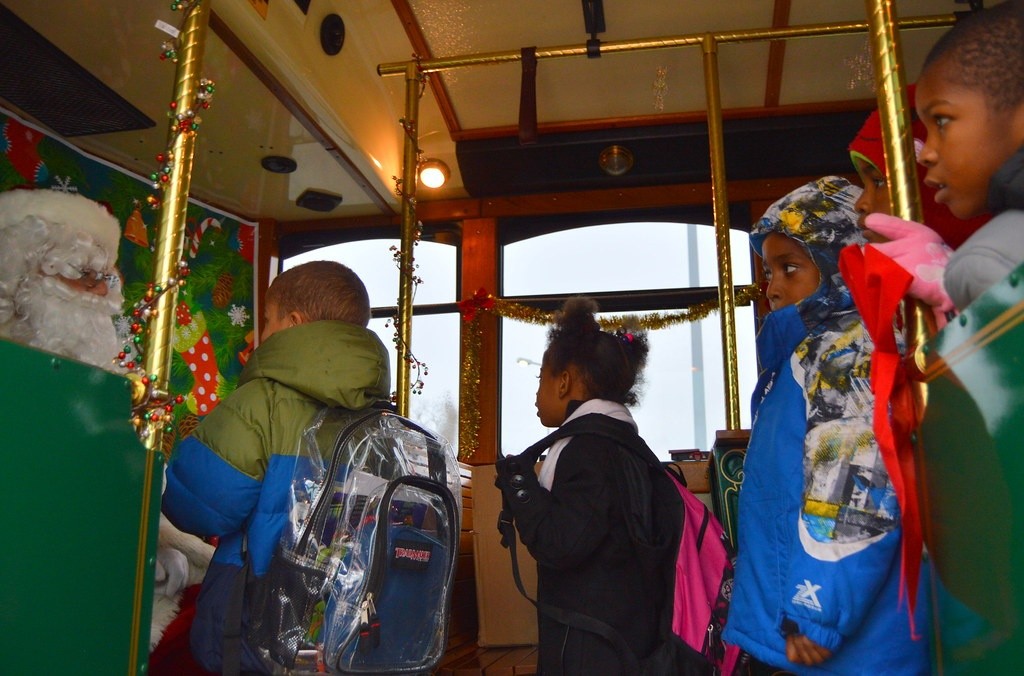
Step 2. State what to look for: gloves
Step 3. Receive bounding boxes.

[860,212,960,317]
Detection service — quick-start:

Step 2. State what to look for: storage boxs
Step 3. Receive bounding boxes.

[469,462,544,649]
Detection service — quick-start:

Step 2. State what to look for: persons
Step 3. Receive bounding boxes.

[2,186,215,676]
[915,0,1023,312]
[501,293,670,676]
[160,260,426,676]
[847,83,997,333]
[720,173,928,674]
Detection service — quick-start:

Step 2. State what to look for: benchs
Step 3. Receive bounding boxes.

[403,460,540,676]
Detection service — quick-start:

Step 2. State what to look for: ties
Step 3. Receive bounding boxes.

[837,244,923,640]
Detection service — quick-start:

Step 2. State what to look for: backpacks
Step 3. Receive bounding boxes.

[495,412,741,676]
[268,399,463,676]
